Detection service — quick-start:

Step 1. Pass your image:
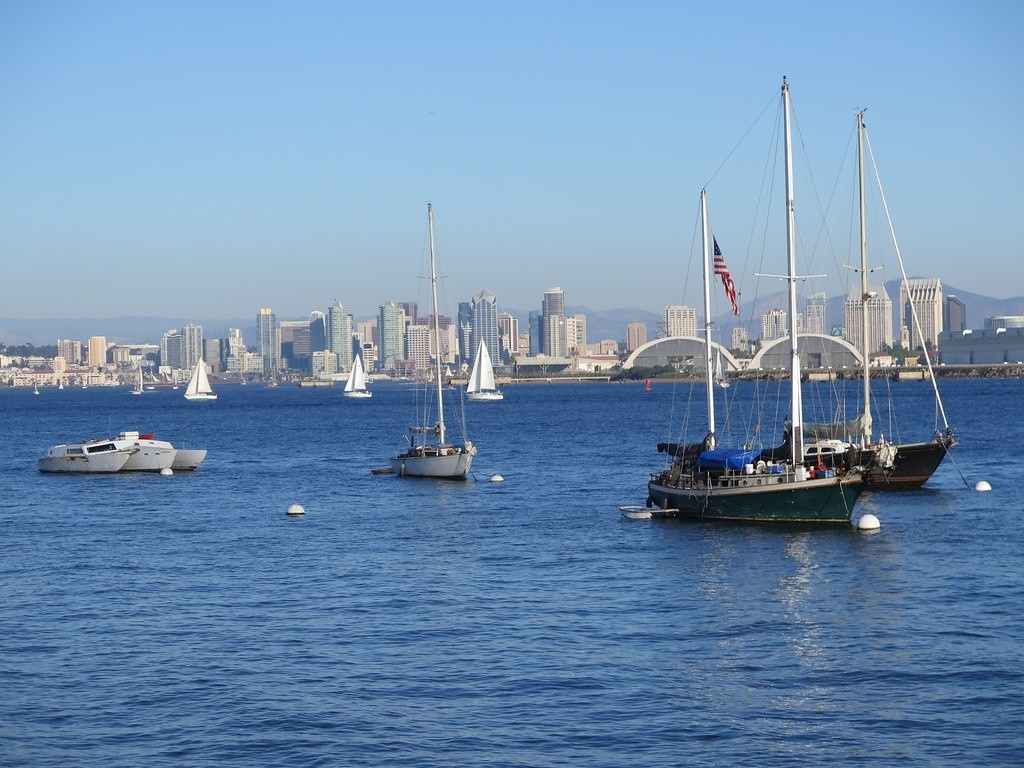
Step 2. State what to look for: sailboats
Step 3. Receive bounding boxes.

[59,383,64,390]
[645,81,876,541]
[341,353,373,398]
[779,105,962,494]
[33,382,39,395]
[464,337,504,401]
[185,357,218,400]
[394,201,478,481]
[131,367,144,395]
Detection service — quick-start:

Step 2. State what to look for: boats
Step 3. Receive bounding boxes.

[36,415,208,477]
[621,505,683,521]
[146,386,155,390]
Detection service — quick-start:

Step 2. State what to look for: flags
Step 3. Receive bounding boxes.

[713,237,741,316]
